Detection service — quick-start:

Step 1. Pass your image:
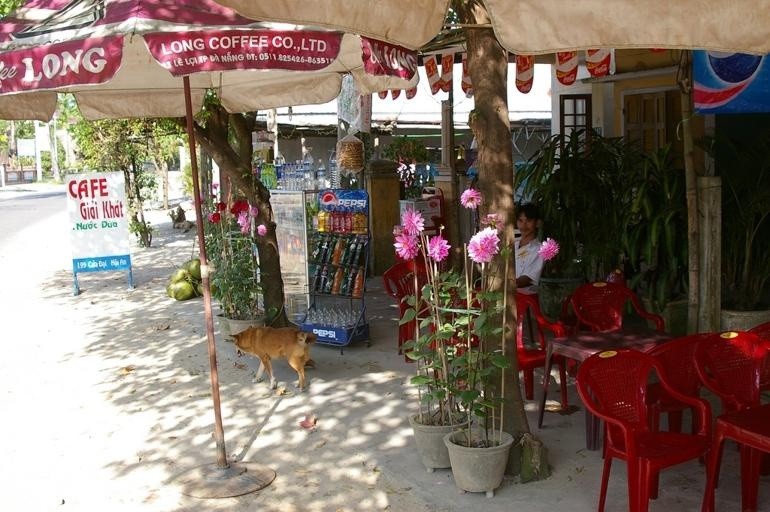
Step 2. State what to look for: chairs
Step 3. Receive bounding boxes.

[575,349,713,510]
[393,186,447,271]
[561,281,667,337]
[697,332,766,511]
[463,283,571,414]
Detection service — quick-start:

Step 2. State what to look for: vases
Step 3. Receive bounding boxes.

[408,412,474,472]
[215,314,231,340]
[226,314,265,336]
[443,429,514,499]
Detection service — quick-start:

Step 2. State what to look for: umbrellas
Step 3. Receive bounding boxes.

[211,3,770,75]
[0,0,421,503]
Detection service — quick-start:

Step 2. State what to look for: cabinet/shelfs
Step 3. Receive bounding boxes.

[300,189,371,356]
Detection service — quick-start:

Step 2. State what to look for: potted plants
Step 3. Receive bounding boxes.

[619,127,690,333]
[713,125,770,332]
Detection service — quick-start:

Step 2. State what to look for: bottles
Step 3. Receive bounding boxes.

[261,151,341,191]
[305,198,368,234]
[310,233,368,298]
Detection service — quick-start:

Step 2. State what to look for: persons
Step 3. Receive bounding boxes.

[511,202,549,290]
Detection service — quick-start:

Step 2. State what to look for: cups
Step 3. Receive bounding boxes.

[304,306,363,329]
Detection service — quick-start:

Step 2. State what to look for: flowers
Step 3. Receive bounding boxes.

[392,205,470,426]
[447,189,558,445]
[396,163,439,200]
[201,183,267,318]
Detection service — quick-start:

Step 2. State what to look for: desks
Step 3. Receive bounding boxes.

[535,334,686,451]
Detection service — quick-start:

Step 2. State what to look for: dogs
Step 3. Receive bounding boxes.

[228,325,317,393]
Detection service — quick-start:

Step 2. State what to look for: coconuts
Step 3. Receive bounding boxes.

[165,259,217,301]
